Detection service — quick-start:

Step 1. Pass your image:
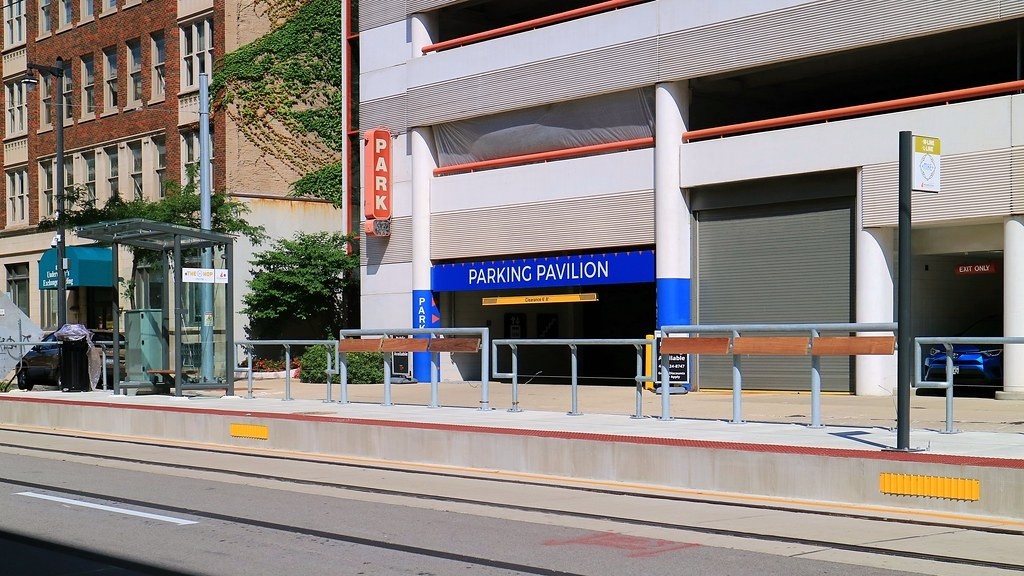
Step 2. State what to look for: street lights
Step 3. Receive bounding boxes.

[20,54,67,331]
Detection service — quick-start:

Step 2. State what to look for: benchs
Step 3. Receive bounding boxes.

[136,370,197,395]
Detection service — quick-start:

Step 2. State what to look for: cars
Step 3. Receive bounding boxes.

[924,316,1004,392]
[15,327,128,391]
